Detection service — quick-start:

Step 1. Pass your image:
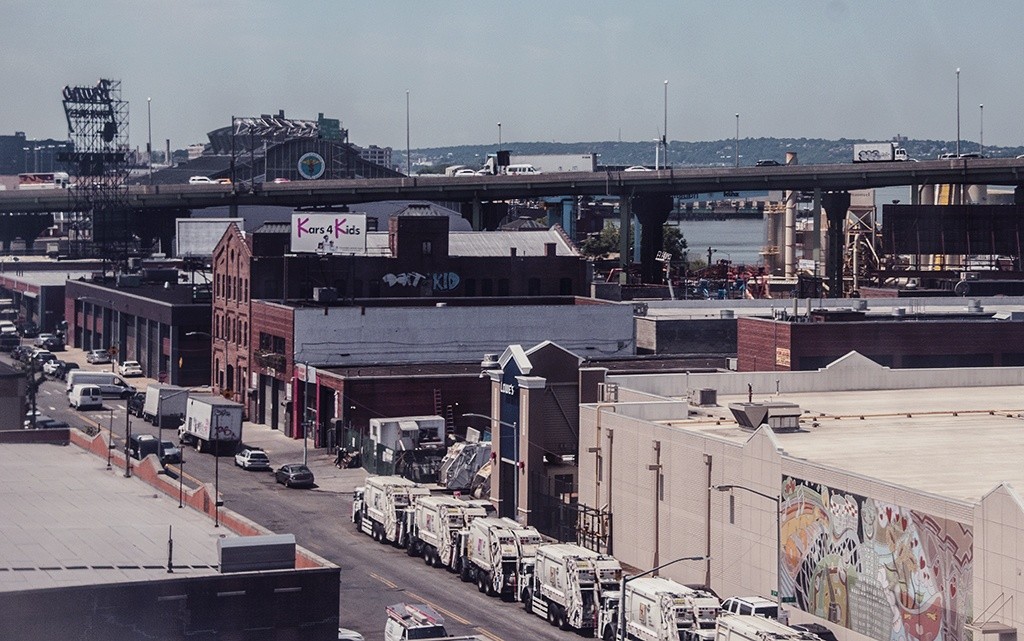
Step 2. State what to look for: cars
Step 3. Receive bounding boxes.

[338,627,366,641]
[159,440,183,464]
[234,447,271,470]
[87,348,111,365]
[0,319,79,381]
[275,464,314,488]
[118,360,143,377]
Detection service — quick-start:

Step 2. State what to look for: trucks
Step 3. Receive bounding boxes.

[142,383,190,426]
[177,393,245,451]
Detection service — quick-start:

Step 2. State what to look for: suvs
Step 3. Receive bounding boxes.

[792,621,840,641]
[719,595,789,625]
[128,392,145,418]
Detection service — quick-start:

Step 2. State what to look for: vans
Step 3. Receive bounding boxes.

[383,617,455,641]
[69,384,103,410]
[125,434,168,468]
[66,369,137,400]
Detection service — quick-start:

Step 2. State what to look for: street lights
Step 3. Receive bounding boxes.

[709,484,784,622]
[261,352,308,466]
[184,331,228,395]
[461,412,519,522]
[619,556,705,641]
